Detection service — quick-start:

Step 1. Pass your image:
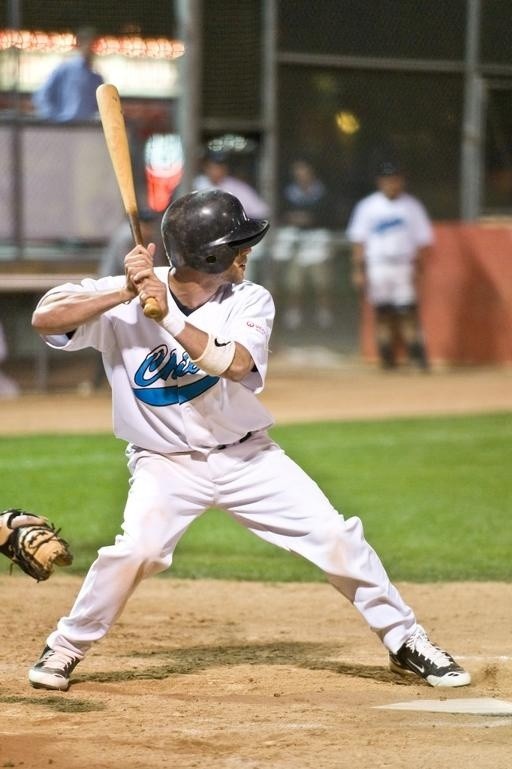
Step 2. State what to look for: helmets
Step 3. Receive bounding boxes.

[161,188,271,275]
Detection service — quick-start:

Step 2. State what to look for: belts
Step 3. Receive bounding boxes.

[214,431,252,451]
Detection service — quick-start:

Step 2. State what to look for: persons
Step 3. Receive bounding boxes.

[33,25,109,125]
[77,202,172,390]
[192,146,267,278]
[342,159,437,375]
[273,155,336,331]
[27,192,474,691]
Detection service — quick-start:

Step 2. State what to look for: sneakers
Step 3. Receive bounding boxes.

[26,643,81,691]
[386,627,473,689]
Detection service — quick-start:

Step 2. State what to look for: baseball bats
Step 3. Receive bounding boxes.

[96,83,161,318]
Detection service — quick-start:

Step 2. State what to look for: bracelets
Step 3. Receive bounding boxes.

[351,260,364,273]
[159,312,185,337]
